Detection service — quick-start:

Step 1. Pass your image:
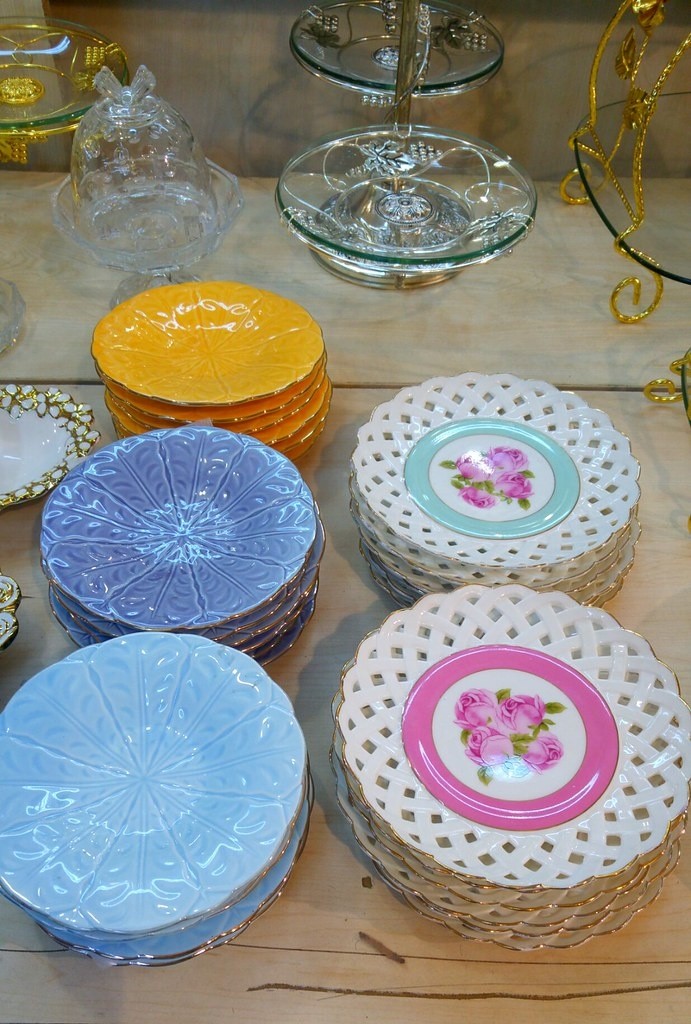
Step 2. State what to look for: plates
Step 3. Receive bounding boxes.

[347,371,639,606]
[1,573,20,652]
[332,584,690,953]
[0,631,316,966]
[39,427,326,671]
[1,277,26,352]
[91,281,333,463]
[2,382,99,508]
